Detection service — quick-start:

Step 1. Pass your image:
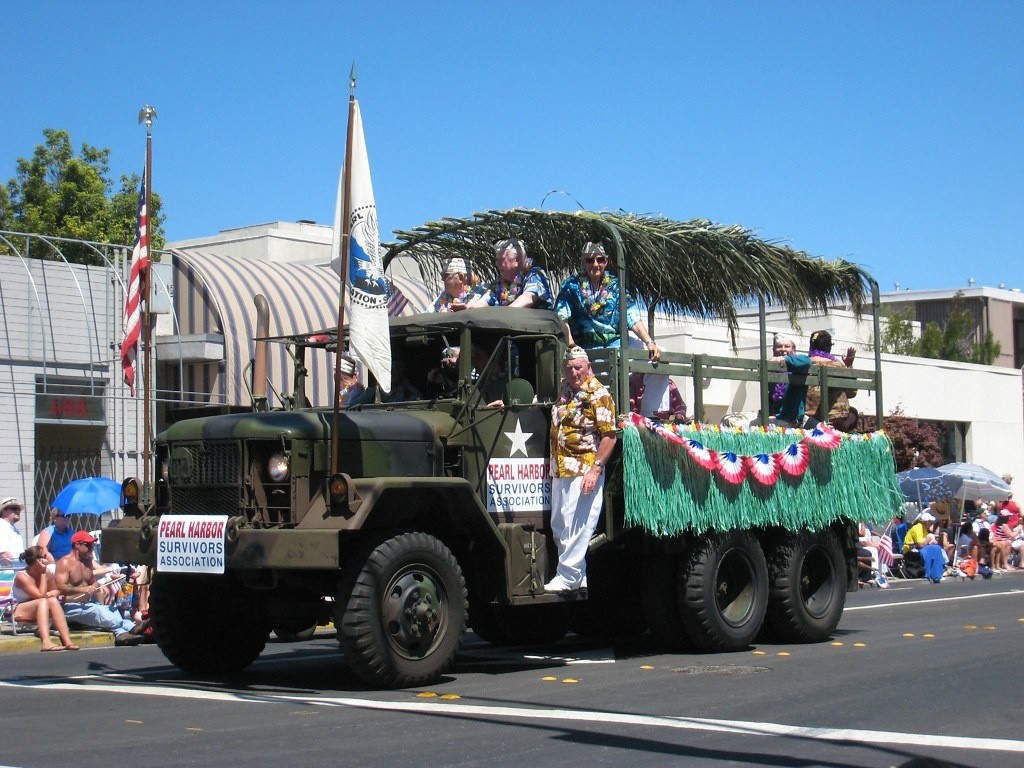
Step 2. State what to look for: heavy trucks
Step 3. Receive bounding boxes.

[98,209,907,690]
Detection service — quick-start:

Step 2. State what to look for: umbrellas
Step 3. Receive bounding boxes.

[51,475,126,516]
[891,467,964,503]
[934,461,1013,569]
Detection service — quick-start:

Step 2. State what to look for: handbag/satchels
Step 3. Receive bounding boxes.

[960,558,977,577]
[898,550,926,579]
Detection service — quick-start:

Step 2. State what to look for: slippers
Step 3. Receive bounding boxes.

[41,646,63,651]
[64,644,78,650]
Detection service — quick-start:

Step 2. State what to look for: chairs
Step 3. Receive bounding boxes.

[896,524,924,578]
[0,569,66,636]
[481,378,534,410]
[872,535,907,580]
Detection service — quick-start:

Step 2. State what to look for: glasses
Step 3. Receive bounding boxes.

[585,256,606,263]
[36,554,46,559]
[79,542,92,548]
[55,514,71,518]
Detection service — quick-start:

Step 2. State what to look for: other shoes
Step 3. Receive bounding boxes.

[543,577,587,591]
[115,632,143,646]
[129,622,149,635]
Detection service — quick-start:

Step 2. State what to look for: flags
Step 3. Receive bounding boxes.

[330,98,410,395]
[118,143,152,399]
[878,520,893,566]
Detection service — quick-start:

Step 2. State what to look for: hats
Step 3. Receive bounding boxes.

[1002,473,1014,479]
[71,530,98,544]
[810,330,835,347]
[1000,509,1013,517]
[919,512,935,522]
[0,497,24,516]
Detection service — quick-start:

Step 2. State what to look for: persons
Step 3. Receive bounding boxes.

[857,522,880,585]
[0,495,149,651]
[902,513,944,584]
[345,343,509,407]
[552,242,672,423]
[450,238,555,313]
[766,331,811,428]
[806,330,860,435]
[913,499,1024,572]
[423,256,500,313]
[486,344,618,592]
[1001,472,1013,485]
[717,411,750,428]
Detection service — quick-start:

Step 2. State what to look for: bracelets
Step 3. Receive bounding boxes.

[646,339,657,345]
[592,460,604,468]
